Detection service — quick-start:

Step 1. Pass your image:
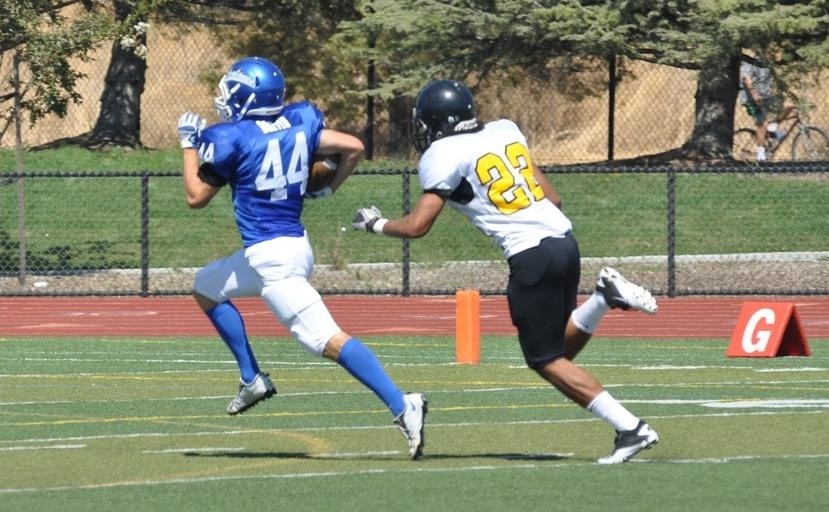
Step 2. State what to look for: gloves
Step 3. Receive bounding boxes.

[352,204,389,237]
[175,110,207,150]
[303,184,334,200]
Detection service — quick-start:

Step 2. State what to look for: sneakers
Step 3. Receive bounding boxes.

[595,418,658,465]
[390,393,428,461]
[595,266,661,317]
[227,370,277,416]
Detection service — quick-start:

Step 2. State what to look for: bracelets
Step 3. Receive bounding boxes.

[371,218,390,235]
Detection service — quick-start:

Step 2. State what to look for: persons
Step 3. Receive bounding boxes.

[175,56,429,461]
[352,78,662,466]
[739,41,798,162]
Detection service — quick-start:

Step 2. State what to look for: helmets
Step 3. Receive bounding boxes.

[215,56,287,121]
[411,78,483,138]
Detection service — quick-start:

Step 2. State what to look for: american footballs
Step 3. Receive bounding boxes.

[306,154,342,192]
